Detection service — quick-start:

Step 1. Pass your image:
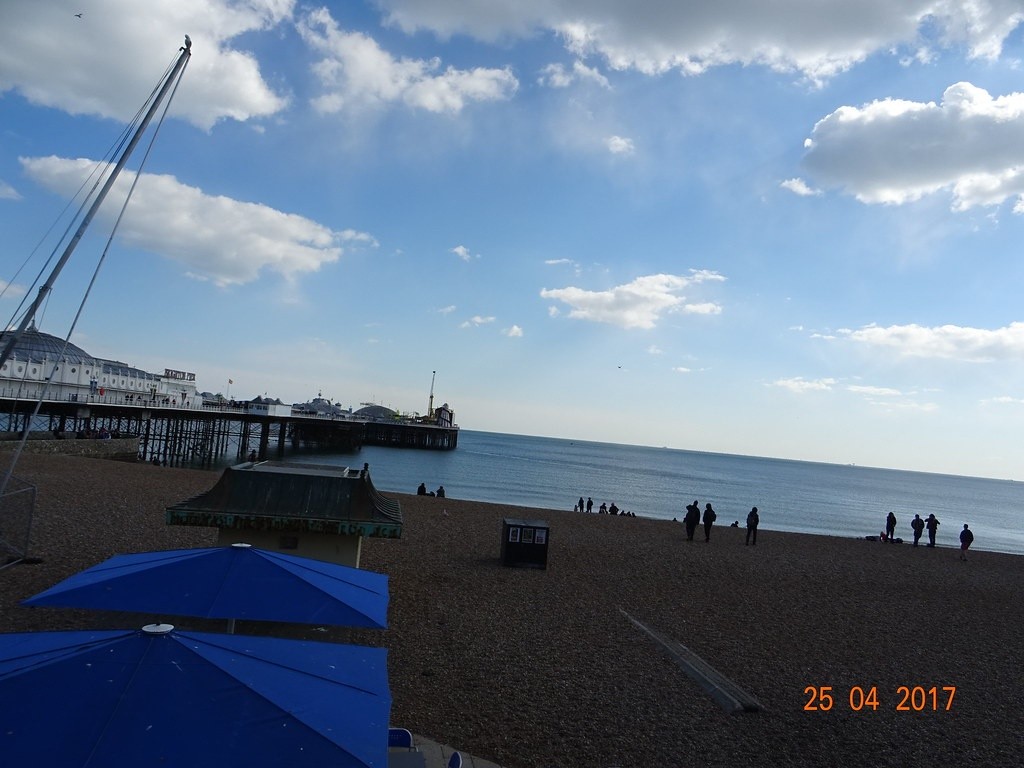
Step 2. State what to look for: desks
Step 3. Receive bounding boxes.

[388,751,425,767]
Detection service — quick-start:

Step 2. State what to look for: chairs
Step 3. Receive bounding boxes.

[447,750,462,768]
[388,727,417,751]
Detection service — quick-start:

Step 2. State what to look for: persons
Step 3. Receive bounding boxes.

[75,428,120,440]
[959,524,973,560]
[417,483,426,496]
[686,500,700,541]
[746,507,759,546]
[731,521,738,527]
[574,497,593,513]
[599,503,637,518]
[880,512,896,544]
[153,456,169,467]
[703,503,716,543]
[911,514,924,547]
[925,514,940,545]
[437,486,445,498]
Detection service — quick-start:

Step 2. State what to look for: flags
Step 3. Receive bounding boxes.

[229,379,232,384]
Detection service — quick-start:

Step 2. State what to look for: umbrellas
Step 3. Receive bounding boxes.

[20,543,388,634]
[0,621,392,768]
